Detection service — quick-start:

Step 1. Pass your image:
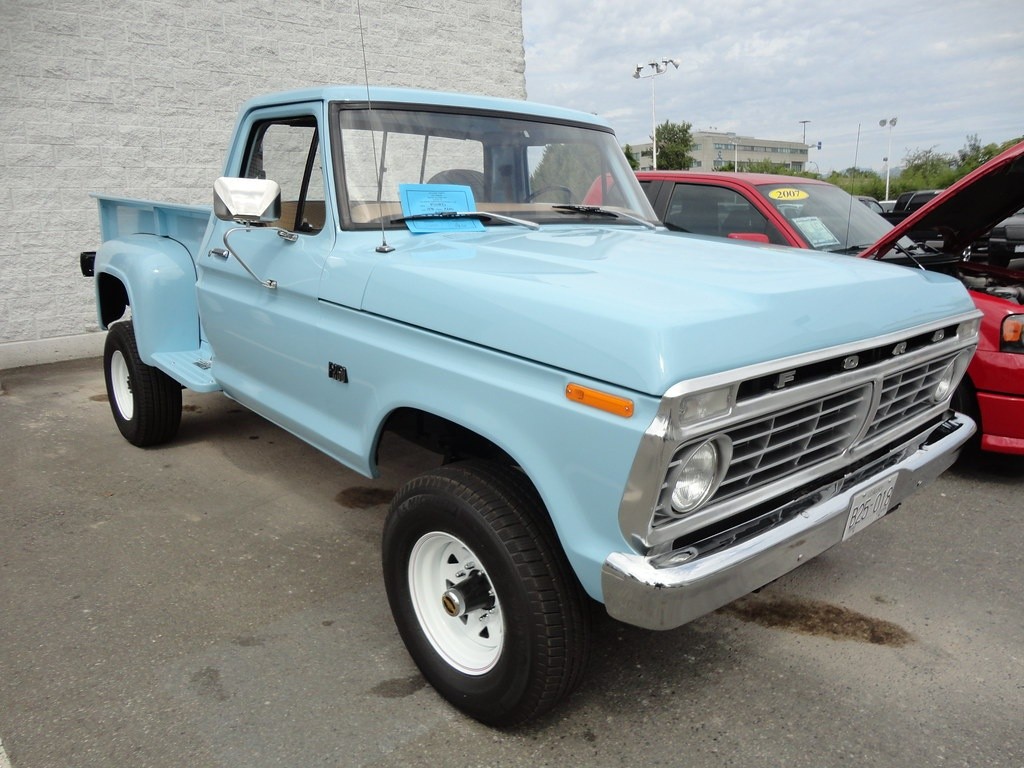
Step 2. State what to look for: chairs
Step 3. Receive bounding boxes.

[428,169,485,206]
[721,210,750,240]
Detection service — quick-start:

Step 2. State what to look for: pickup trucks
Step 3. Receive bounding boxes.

[79,85,984,732]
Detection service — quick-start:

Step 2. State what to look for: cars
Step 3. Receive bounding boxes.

[577,137,1024,464]
[850,187,1024,272]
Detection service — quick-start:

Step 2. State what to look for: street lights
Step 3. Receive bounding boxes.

[798,120,811,144]
[632,57,680,170]
[878,117,898,201]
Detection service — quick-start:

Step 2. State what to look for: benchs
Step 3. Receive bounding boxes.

[268,200,405,230]
